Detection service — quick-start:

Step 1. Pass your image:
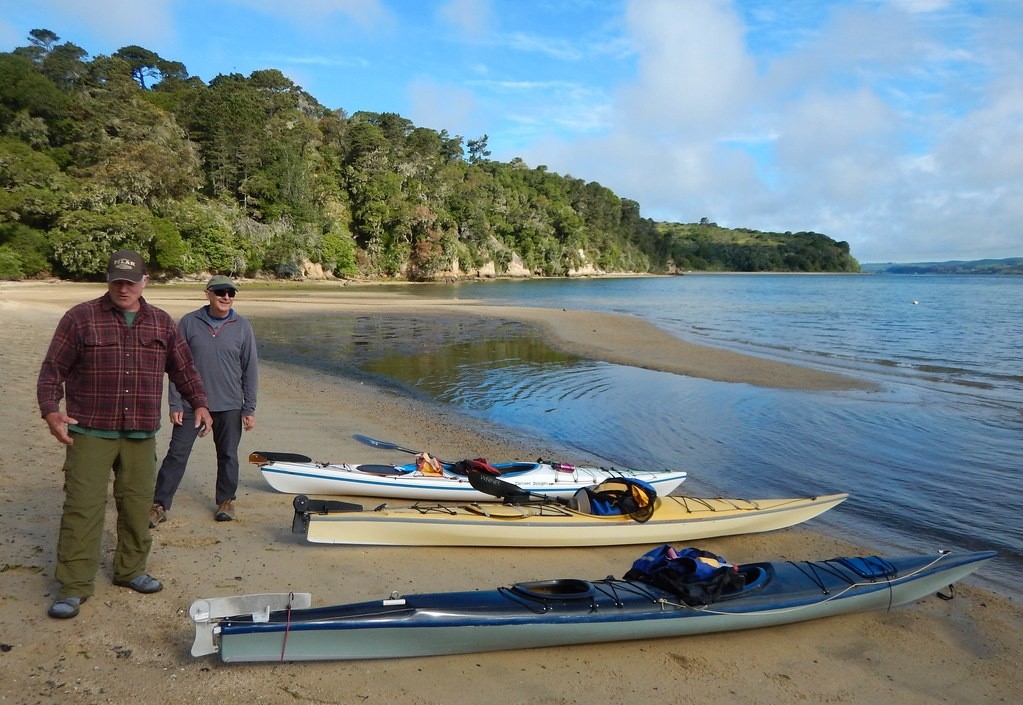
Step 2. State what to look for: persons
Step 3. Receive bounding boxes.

[37,251,214,620]
[150,275,258,528]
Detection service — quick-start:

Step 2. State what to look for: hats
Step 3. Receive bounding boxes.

[206,274,239,292]
[107,249,144,283]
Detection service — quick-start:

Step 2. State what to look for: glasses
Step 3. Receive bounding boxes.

[210,288,235,297]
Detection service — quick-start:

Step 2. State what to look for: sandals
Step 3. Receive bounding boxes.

[48,597,87,618]
[114,574,164,594]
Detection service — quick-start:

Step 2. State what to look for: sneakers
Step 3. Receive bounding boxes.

[215,499,235,521]
[149,501,166,528]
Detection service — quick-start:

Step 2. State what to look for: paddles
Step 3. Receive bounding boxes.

[469,471,566,504]
[352,434,456,466]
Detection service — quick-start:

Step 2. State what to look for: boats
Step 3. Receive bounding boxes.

[292,493,851,548]
[249,451,687,504]
[186,549,998,663]
